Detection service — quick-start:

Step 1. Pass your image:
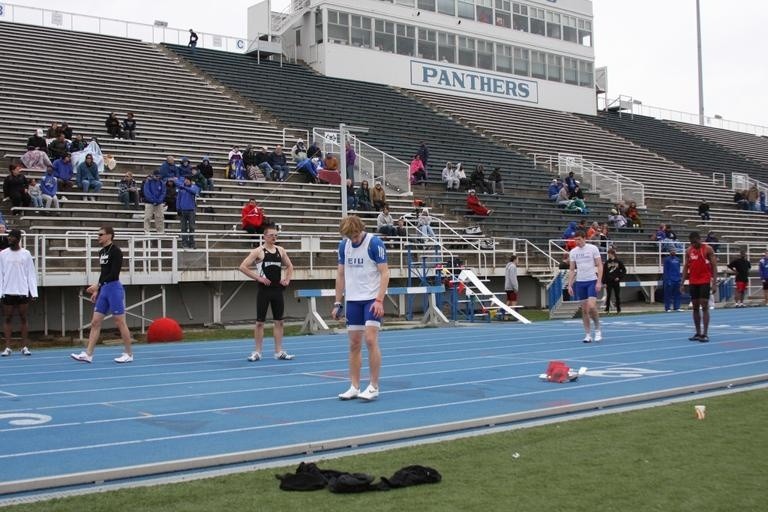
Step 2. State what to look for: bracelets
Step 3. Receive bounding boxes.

[334,302,341,306]
[376,297,384,304]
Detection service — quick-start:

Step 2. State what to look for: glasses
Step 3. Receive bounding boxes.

[99,233,105,236]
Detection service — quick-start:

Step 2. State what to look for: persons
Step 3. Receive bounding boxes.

[703,229,726,248]
[336,175,388,209]
[696,199,719,223]
[373,202,441,248]
[679,232,717,342]
[70,225,135,364]
[607,197,648,233]
[239,224,295,361]
[549,170,591,214]
[228,131,359,183]
[560,218,610,253]
[331,216,390,400]
[733,186,766,212]
[568,230,604,343]
[653,223,680,253]
[242,198,281,232]
[411,138,506,217]
[188,29,198,53]
[505,248,768,315]
[1,108,217,251]
[0,228,39,357]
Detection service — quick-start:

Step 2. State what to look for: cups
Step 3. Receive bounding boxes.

[232,225,236,231]
[693,404,707,421]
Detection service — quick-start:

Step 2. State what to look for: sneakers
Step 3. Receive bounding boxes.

[338,385,360,400]
[583,335,593,343]
[21,346,31,355]
[357,385,379,400]
[1,347,12,356]
[247,352,262,361]
[114,353,133,363]
[71,351,93,363]
[273,351,295,360]
[594,330,601,341]
[666,309,708,342]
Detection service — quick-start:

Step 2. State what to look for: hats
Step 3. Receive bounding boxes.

[9,230,21,240]
